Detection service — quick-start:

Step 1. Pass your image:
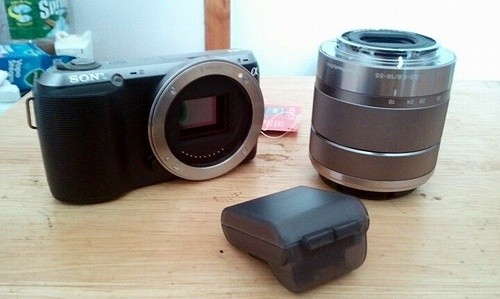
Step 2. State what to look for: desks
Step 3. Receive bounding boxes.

[0,75,500,299]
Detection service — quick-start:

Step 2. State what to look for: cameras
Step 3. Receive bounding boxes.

[33,49,265,204]
